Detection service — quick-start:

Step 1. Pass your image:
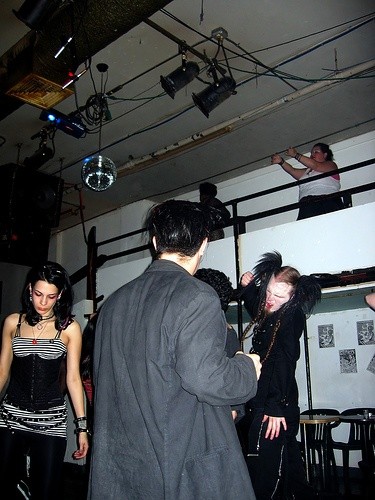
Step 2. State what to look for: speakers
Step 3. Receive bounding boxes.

[0,163,63,266]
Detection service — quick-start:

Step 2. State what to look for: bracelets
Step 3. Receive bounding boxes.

[74,417,88,433]
[279,159,284,165]
[295,153,301,160]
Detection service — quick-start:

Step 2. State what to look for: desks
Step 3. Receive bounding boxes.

[339,416,375,475]
[300,415,338,488]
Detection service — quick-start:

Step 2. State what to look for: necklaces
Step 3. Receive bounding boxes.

[241,310,279,366]
[32,313,55,344]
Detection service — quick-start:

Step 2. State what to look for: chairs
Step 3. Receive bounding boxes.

[301,409,340,471]
[324,407,375,480]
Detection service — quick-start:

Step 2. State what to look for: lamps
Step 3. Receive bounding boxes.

[23,135,54,171]
[82,98,117,190]
[159,46,200,100]
[191,65,236,118]
[38,107,87,140]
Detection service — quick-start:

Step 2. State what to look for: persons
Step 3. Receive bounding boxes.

[0,261,89,500]
[271,142,341,220]
[81,199,262,500]
[198,182,231,242]
[191,267,245,422]
[238,248,322,500]
